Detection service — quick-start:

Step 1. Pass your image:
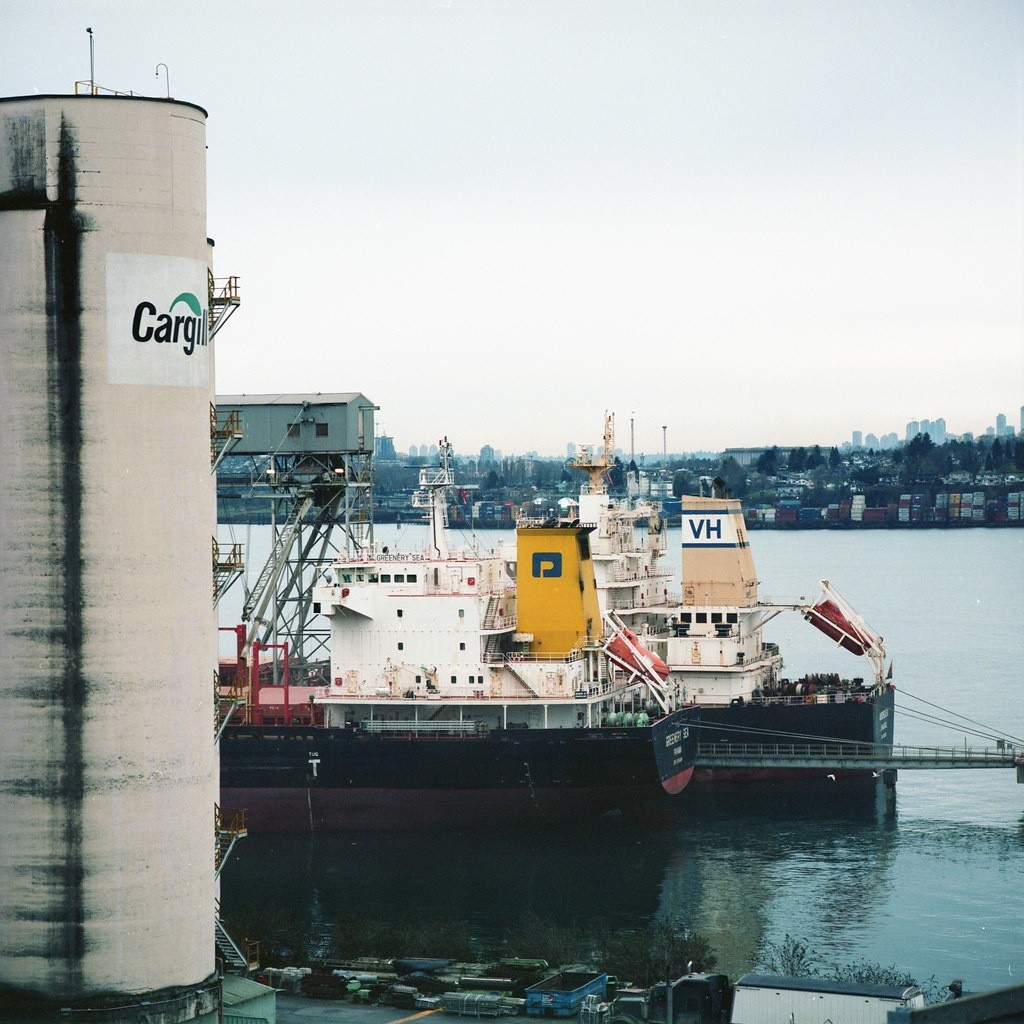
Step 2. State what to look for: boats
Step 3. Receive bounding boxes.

[219,414,896,817]
